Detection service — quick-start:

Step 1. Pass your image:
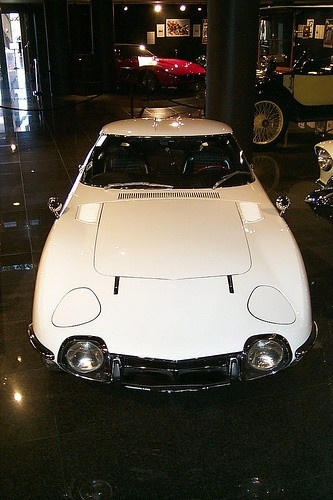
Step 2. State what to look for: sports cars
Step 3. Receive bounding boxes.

[26,116,319,393]
[70,43,206,101]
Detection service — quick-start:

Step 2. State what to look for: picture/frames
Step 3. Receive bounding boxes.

[146,18,201,45]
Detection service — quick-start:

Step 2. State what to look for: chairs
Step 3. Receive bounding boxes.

[275,50,306,75]
[182,152,232,175]
[111,156,149,175]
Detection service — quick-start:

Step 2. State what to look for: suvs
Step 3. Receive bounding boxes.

[253,5,332,151]
